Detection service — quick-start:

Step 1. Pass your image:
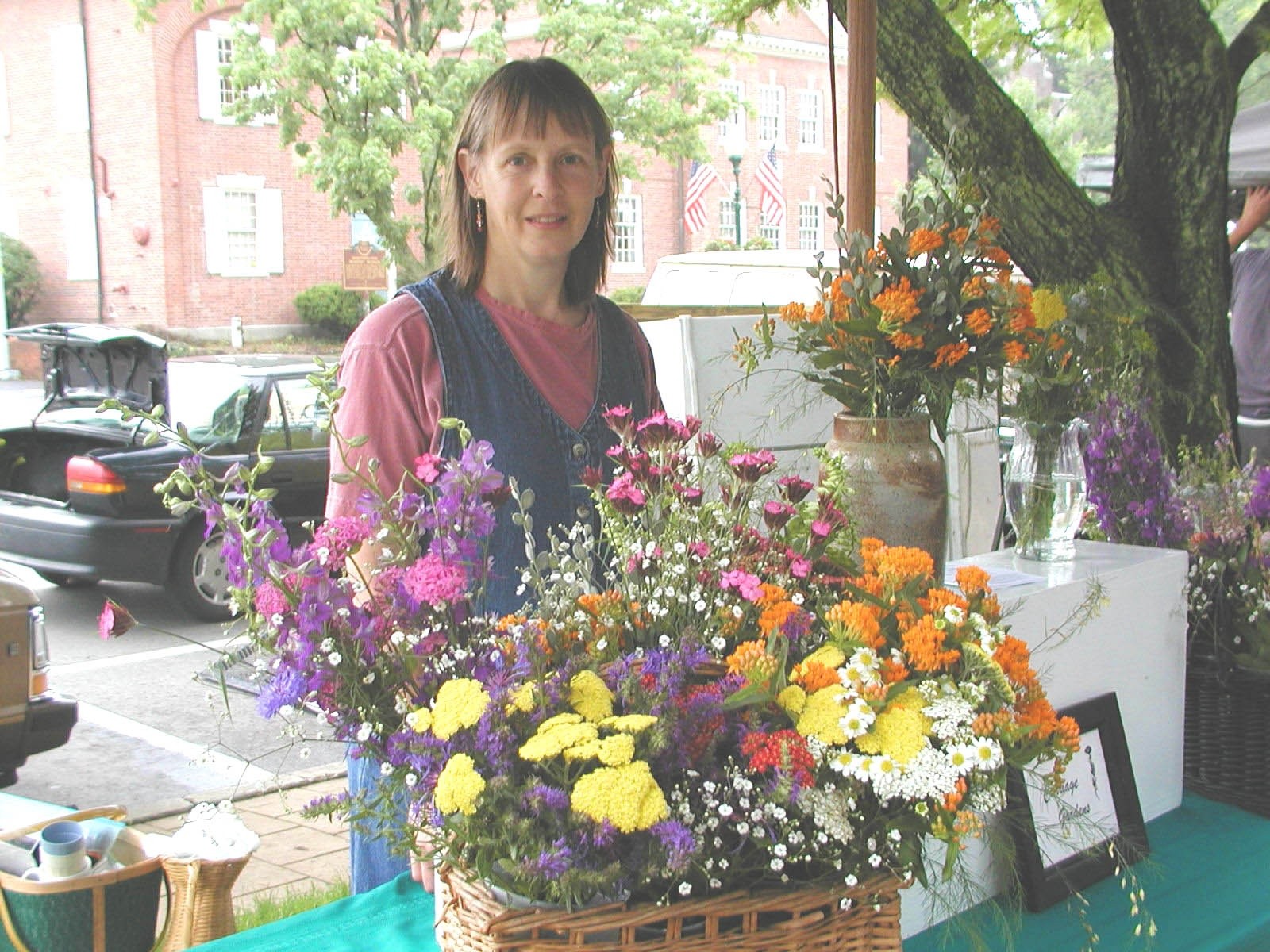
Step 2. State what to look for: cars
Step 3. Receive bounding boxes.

[0,322,330,619]
[0,558,82,789]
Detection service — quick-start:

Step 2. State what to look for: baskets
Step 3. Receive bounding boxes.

[1187,649,1269,820]
[433,841,901,952]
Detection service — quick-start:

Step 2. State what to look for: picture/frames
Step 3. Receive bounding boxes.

[1003,692,1152,912]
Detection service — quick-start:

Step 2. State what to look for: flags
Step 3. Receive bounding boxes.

[681,156,717,234]
[755,143,785,226]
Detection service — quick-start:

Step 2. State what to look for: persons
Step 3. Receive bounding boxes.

[1226,186,1270,480]
[323,57,669,910]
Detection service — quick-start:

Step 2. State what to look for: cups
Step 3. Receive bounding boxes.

[22,821,92,885]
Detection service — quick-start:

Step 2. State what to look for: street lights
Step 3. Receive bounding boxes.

[724,133,746,250]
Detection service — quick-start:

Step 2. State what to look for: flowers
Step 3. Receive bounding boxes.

[99,118,1269,916]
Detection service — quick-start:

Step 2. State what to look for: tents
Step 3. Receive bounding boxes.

[1075,100,1270,220]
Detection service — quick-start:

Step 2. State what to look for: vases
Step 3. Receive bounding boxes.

[818,410,950,594]
[1003,420,1089,560]
